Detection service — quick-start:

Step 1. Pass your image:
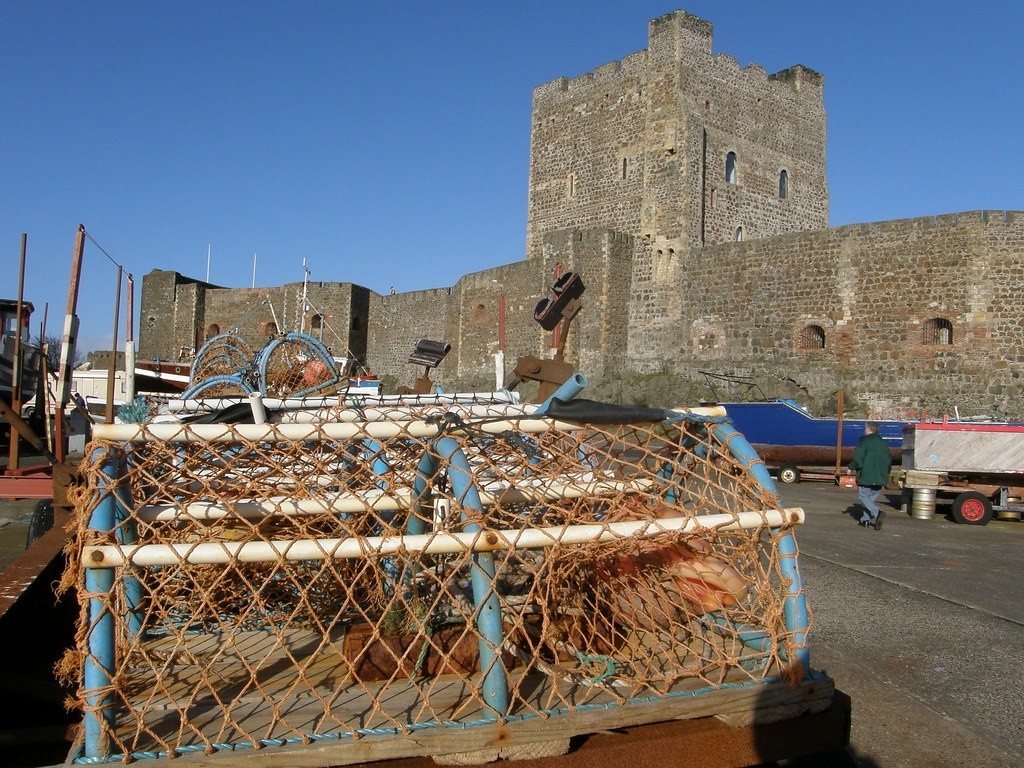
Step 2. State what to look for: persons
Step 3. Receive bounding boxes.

[848,420,892,530]
[74,393,86,409]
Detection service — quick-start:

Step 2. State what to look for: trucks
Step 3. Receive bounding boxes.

[901,409,1024,525]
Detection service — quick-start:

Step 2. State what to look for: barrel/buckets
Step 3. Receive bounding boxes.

[911,488,937,520]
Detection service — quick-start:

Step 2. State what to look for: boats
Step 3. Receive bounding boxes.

[697,370,995,468]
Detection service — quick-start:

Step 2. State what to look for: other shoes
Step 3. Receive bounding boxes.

[875,510,885,530]
[858,520,869,528]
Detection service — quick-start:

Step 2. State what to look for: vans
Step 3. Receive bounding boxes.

[21,370,128,418]
